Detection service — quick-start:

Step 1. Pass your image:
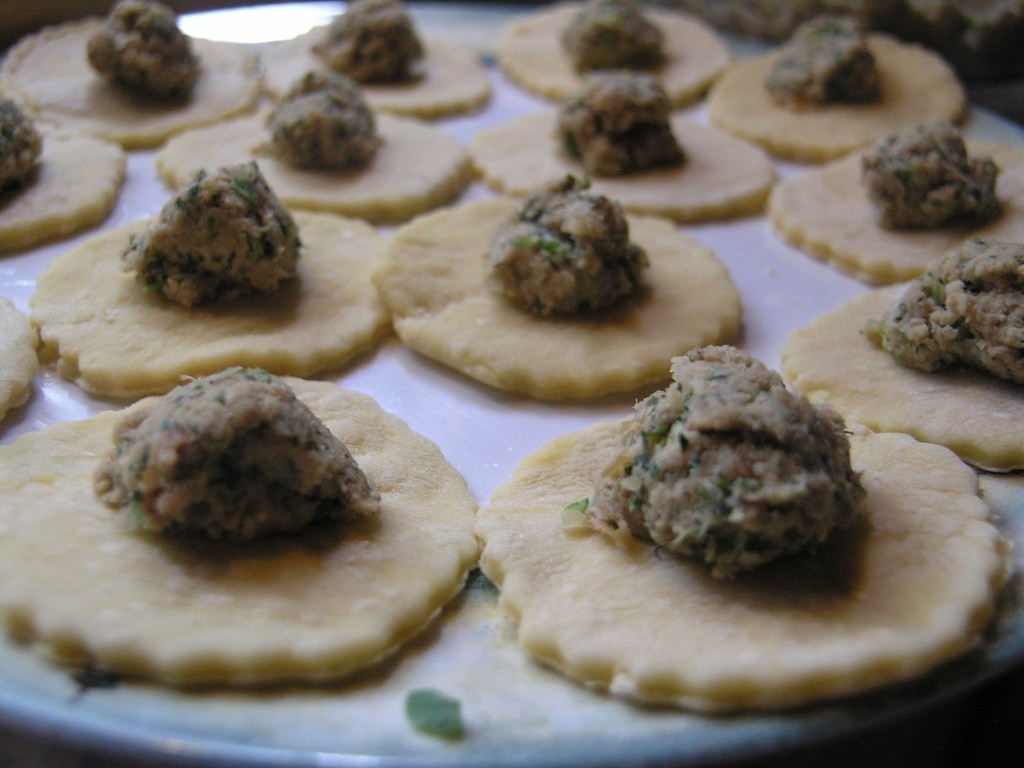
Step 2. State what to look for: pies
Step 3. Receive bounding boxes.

[1,2,1024,708]
